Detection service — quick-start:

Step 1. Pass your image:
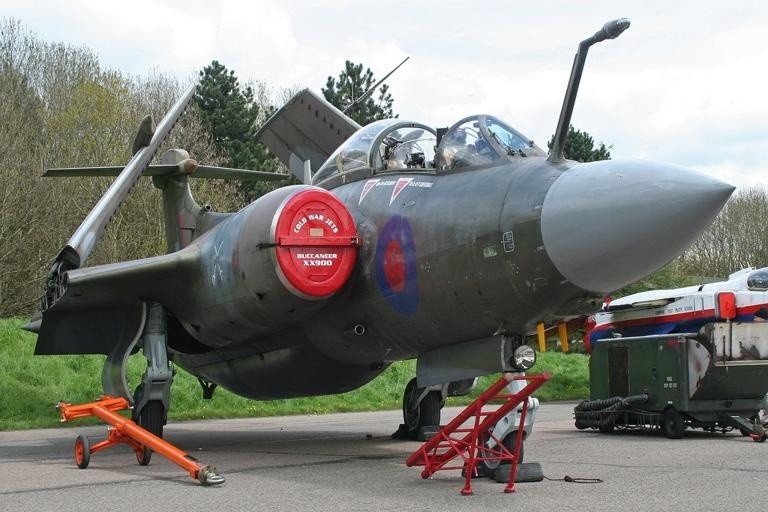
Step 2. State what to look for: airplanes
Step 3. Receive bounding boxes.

[16,14,738,476]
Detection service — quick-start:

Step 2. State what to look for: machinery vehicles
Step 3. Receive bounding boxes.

[571,263,768,443]
